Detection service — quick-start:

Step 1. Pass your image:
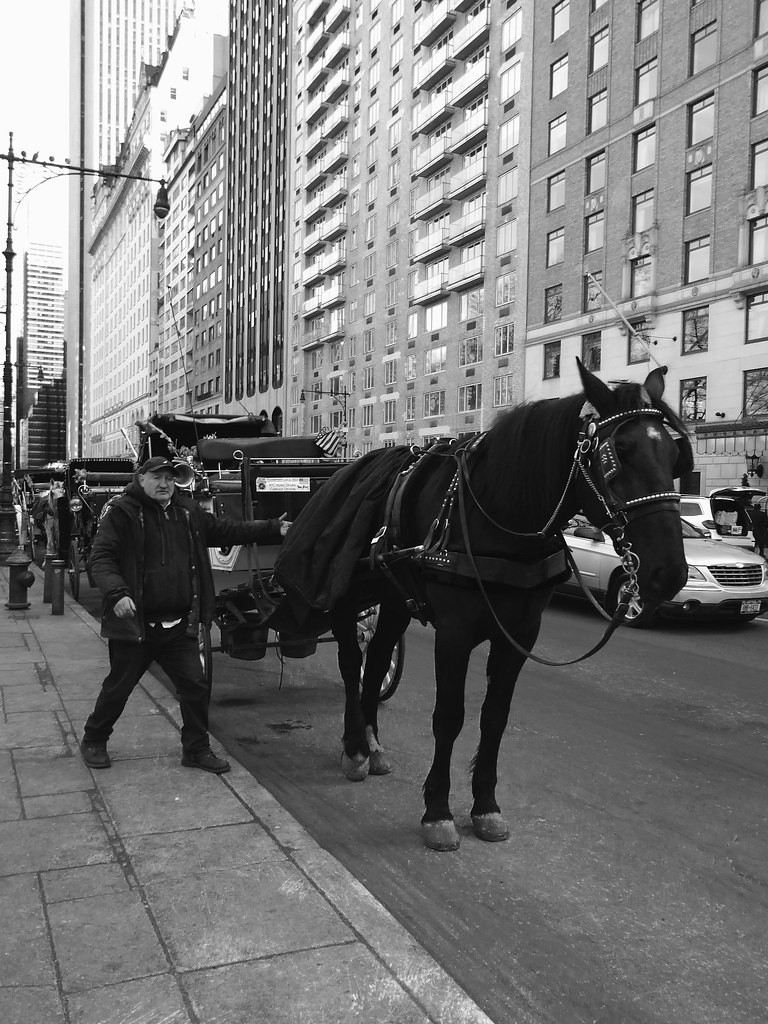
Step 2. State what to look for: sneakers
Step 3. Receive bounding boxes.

[180,741,233,776]
[78,736,115,770]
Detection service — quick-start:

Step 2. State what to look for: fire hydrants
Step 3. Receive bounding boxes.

[6,545,35,610]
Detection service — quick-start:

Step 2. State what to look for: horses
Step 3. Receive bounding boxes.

[329,356,688,850]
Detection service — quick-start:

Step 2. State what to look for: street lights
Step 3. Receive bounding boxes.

[1,132,170,567]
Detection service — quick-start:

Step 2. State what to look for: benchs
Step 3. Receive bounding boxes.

[64,458,136,500]
[135,413,278,514]
[12,467,64,505]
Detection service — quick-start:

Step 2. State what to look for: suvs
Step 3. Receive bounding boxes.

[679,487,766,553]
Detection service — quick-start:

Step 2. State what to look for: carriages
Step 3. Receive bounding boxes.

[13,357,693,852]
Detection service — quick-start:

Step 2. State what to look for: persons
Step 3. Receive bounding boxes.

[79,455,295,773]
[748,503,766,560]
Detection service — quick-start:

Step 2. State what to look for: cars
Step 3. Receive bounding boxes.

[558,512,768,627]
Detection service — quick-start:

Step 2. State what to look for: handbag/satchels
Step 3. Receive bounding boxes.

[715,511,738,526]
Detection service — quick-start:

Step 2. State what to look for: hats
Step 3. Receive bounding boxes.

[140,457,181,477]
